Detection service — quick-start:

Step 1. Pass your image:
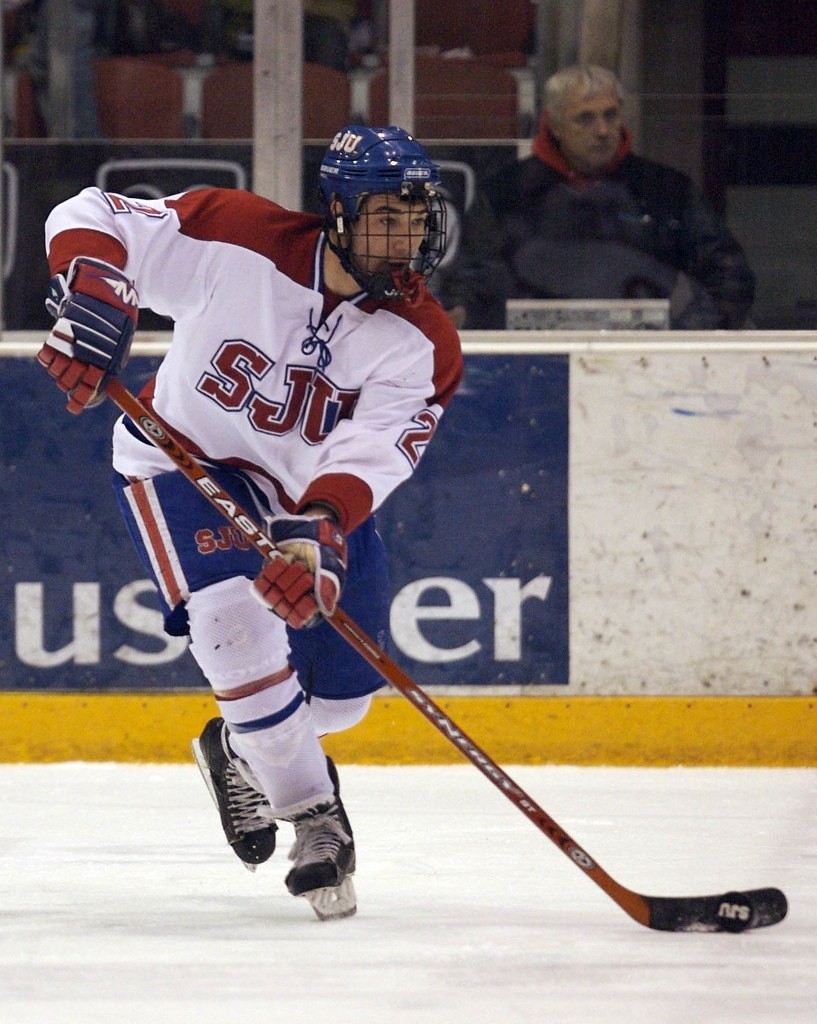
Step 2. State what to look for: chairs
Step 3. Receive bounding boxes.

[1,0,532,137]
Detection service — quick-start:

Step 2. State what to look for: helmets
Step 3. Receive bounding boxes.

[319,124,448,299]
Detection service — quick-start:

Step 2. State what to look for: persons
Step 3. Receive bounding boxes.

[438,66,757,331]
[36,125,464,898]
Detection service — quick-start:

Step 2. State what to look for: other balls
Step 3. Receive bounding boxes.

[713,890,756,934]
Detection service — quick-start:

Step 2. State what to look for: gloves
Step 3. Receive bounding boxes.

[249,513,348,628]
[37,255,138,415]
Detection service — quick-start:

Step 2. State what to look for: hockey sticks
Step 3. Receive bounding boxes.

[101,375,796,938]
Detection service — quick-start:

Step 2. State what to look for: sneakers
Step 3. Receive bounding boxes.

[257,756,357,921]
[191,717,279,873]
